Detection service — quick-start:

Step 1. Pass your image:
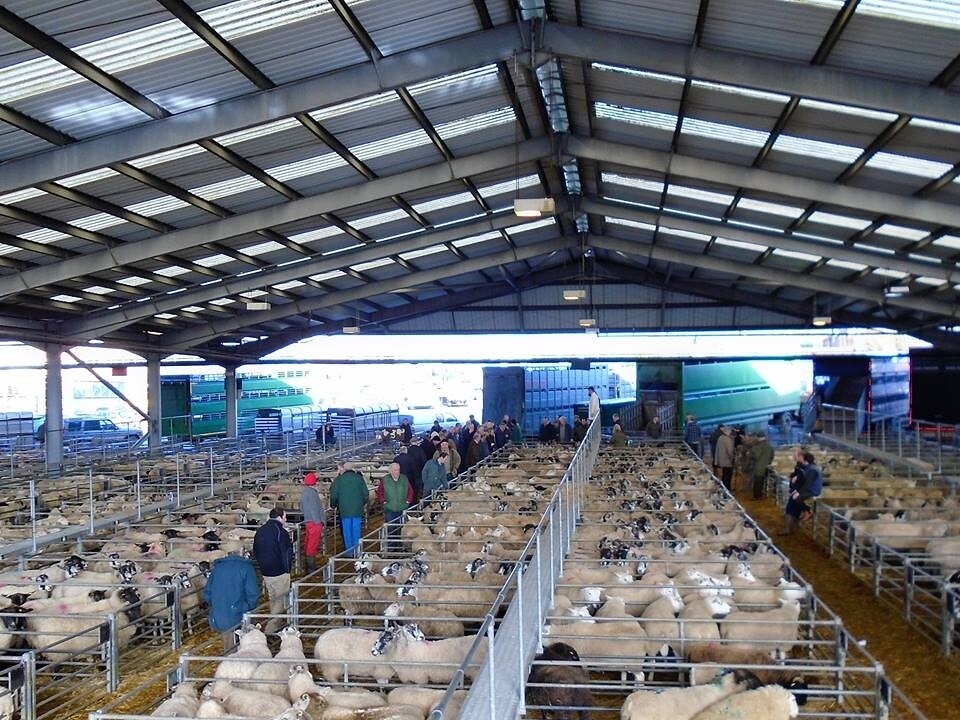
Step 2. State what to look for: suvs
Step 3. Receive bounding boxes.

[36,417,143,459]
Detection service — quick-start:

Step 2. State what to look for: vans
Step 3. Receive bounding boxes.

[381,411,464,438]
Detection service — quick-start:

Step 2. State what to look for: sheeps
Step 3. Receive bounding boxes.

[0,444,959,720]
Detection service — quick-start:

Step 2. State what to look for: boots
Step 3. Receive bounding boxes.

[776,515,793,535]
[749,486,763,501]
[304,557,324,575]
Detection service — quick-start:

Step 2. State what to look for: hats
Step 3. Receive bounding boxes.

[431,431,439,437]
[433,435,440,441]
[304,472,316,485]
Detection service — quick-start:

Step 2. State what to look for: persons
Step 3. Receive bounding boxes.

[203,538,259,652]
[609,424,626,447]
[612,413,623,432]
[588,387,600,424]
[779,453,823,536]
[686,415,699,456]
[316,414,591,511]
[646,415,662,438]
[253,508,293,630]
[789,445,813,521]
[300,473,325,574]
[710,414,774,535]
[377,463,413,556]
[330,462,369,556]
